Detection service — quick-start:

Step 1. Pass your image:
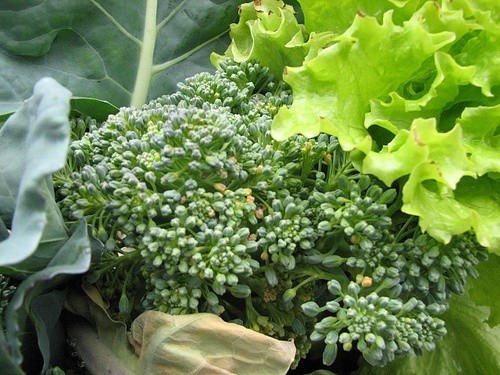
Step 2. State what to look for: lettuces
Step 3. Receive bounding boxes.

[209,0,500,255]
